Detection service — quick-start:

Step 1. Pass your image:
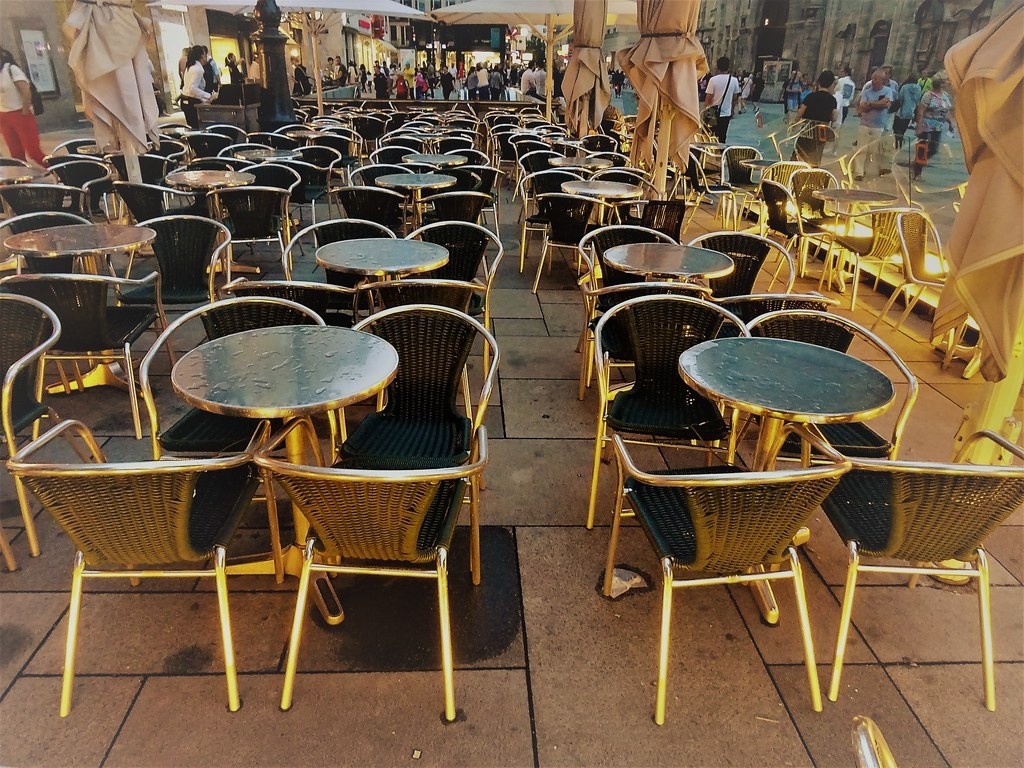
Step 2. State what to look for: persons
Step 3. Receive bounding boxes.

[0,45,50,173]
[697,53,957,185]
[178,37,626,132]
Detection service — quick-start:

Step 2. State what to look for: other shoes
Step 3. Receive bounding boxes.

[855,176,862,182]
[915,175,925,182]
[744,107,747,113]
[879,170,891,176]
[755,107,759,114]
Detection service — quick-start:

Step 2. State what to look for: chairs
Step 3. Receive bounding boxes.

[139,295,339,502]
[586,293,739,532]
[4,420,285,717]
[252,415,481,723]
[348,303,501,586]
[800,421,1024,711]
[737,308,919,466]
[0,293,91,558]
[0,101,1024,419]
[603,422,853,726]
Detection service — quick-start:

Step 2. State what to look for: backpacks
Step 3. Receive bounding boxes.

[203,59,213,84]
[298,67,311,94]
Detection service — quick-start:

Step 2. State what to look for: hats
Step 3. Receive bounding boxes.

[405,60,410,65]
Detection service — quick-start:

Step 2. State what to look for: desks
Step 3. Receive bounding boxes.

[543,137,588,144]
[561,181,644,285]
[315,238,449,311]
[286,130,337,146]
[674,141,728,206]
[677,336,897,628]
[375,173,457,241]
[165,170,261,274]
[0,165,51,220]
[548,157,613,171]
[738,159,788,239]
[6,224,157,398]
[804,189,898,293]
[401,154,468,171]
[170,326,400,630]
[234,149,303,246]
[158,127,211,163]
[76,146,120,220]
[603,242,735,292]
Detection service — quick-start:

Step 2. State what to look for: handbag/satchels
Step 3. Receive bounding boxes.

[8,62,44,116]
[702,104,720,128]
[449,89,458,100]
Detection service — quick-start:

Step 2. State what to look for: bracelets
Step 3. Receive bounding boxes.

[870,100,873,106]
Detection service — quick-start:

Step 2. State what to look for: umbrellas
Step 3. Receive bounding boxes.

[62,0,163,185]
[625,0,705,202]
[146,1,430,113]
[561,0,613,140]
[926,0,1024,472]
[428,0,637,122]
